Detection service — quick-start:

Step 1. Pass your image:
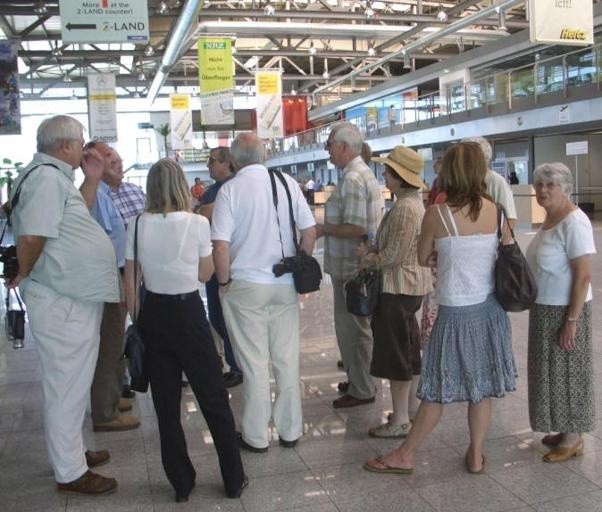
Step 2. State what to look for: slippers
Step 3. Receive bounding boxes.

[362,454,415,475]
[465,448,486,475]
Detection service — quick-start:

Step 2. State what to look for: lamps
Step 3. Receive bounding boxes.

[264,2,275,15]
[137,69,146,82]
[155,1,171,14]
[368,42,374,54]
[32,1,46,13]
[63,68,72,82]
[436,7,449,21]
[144,41,155,56]
[72,90,77,100]
[363,2,375,16]
[308,42,318,54]
[51,48,62,55]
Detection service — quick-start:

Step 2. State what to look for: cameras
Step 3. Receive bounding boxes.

[272,250,307,278]
[0,245,19,283]
[4,310,26,349]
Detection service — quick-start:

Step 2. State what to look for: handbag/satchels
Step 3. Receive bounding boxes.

[344,266,382,317]
[120,324,149,392]
[494,202,538,313]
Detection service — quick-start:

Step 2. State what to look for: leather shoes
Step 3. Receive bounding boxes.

[278,435,299,448]
[56,384,140,496]
[227,476,249,499]
[235,431,268,453]
[224,372,243,388]
[333,381,417,438]
[175,480,195,503]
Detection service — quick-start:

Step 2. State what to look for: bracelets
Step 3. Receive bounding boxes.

[565,315,578,322]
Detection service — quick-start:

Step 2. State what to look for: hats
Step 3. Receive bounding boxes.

[369,145,429,191]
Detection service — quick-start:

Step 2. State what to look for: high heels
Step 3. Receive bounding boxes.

[543,434,561,446]
[542,439,585,464]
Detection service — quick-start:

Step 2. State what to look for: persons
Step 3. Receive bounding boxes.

[525,162,596,463]
[7,114,317,503]
[315,122,517,475]
[388,106,397,129]
